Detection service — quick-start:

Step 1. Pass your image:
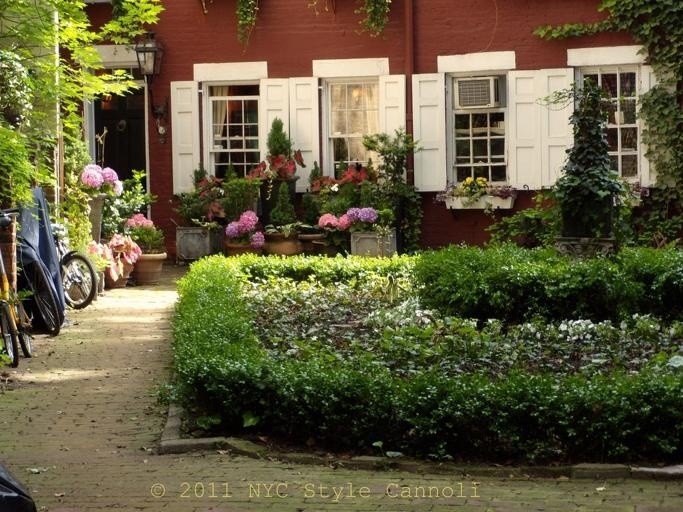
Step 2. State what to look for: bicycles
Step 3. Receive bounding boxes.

[44,200,99,311]
[0,208,34,370]
[13,187,72,339]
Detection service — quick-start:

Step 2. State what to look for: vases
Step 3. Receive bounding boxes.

[104,252,167,288]
[225,241,253,257]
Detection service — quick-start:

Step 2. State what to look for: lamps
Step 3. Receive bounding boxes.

[134,29,168,126]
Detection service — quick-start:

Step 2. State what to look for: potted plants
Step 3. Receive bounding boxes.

[260,116,327,257]
[552,86,617,261]
[168,162,222,266]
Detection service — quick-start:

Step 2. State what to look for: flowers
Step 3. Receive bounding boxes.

[318,206,378,235]
[87,212,165,281]
[80,162,122,197]
[224,209,265,250]
[434,175,518,208]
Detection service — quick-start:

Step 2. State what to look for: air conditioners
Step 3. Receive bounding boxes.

[452,76,501,110]
